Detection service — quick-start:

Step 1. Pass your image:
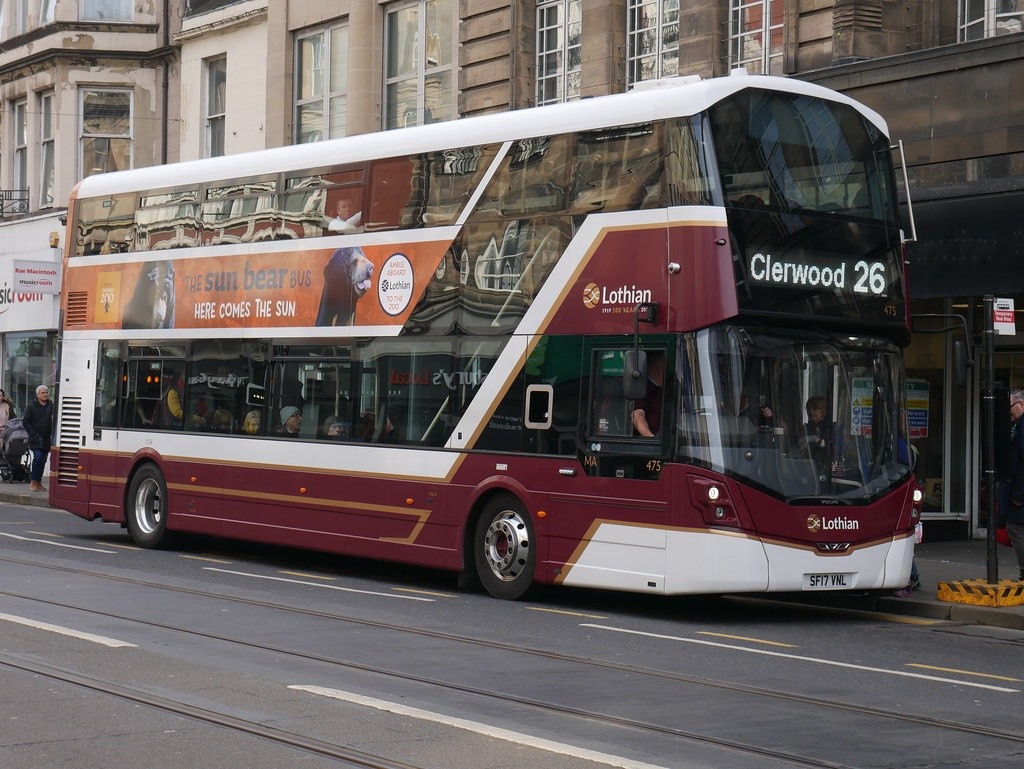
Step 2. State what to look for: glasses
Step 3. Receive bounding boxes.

[290,413,301,418]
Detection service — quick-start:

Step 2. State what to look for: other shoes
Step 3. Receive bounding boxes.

[893,575,921,597]
[30,482,47,491]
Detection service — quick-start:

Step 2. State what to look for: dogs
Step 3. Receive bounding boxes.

[122,261,176,329]
[315,246,374,326]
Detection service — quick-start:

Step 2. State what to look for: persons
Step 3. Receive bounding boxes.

[105,362,395,440]
[22,385,53,492]
[630,357,663,438]
[723,378,774,427]
[998,387,1024,580]
[0,389,17,448]
[891,438,921,597]
[327,195,382,231]
[799,395,844,459]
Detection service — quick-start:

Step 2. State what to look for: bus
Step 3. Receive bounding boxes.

[48,68,978,605]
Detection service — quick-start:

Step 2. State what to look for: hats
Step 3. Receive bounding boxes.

[280,406,300,426]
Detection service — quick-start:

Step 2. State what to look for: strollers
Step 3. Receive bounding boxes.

[0,417,34,484]
